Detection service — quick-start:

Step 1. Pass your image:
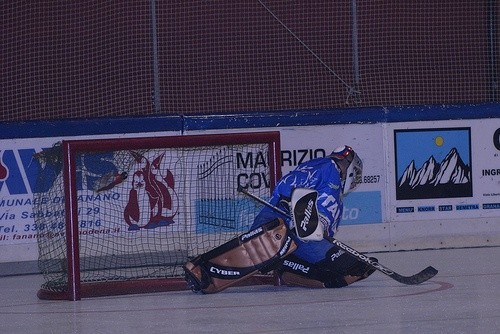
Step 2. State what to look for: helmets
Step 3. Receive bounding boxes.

[328,144,363,163]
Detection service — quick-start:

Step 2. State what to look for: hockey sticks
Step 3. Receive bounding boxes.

[237,184,439,286]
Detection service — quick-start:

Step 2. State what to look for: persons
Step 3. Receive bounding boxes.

[183,145,380,292]
